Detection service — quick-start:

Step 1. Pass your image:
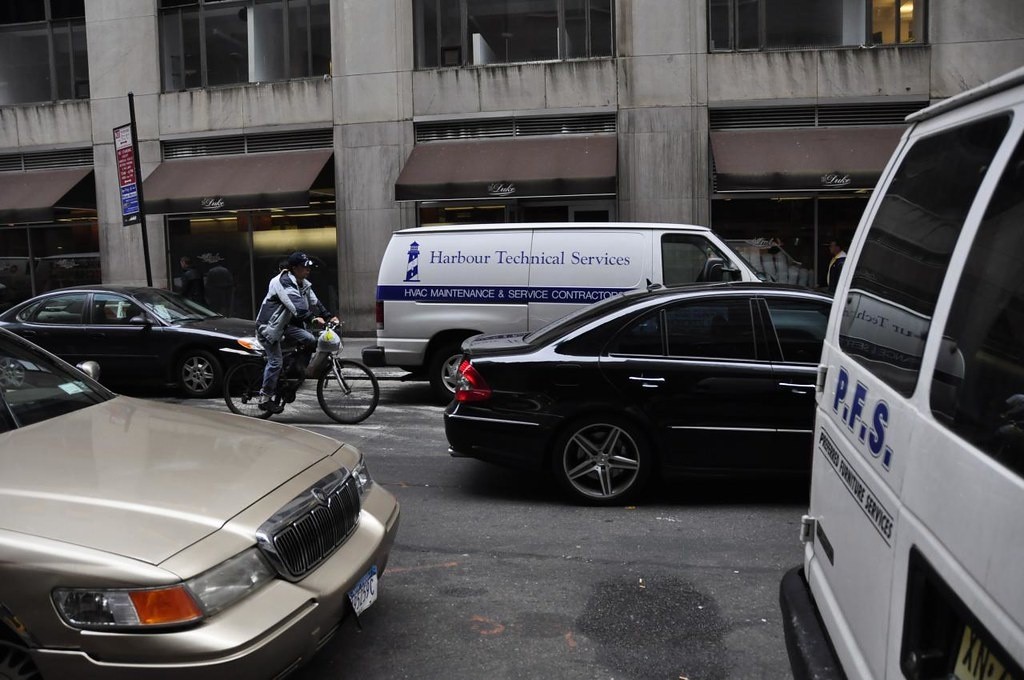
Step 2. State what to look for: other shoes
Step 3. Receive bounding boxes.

[287,364,305,376]
[258,397,284,414]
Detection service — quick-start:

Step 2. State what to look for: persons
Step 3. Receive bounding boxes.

[255,251,339,414]
[180,256,203,303]
[203,256,233,315]
[826,240,847,296]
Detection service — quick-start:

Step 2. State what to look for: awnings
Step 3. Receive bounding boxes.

[0,166,94,224]
[142,148,334,214]
[395,132,618,208]
[710,125,909,194]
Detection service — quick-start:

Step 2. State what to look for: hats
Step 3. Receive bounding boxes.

[288,252,313,268]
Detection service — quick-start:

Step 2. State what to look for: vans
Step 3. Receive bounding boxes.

[724,239,815,288]
[361,222,782,407]
[840,288,966,426]
[778,67,1024,680]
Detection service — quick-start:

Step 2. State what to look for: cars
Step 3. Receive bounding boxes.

[0,326,402,680]
[0,283,290,399]
[444,280,834,508]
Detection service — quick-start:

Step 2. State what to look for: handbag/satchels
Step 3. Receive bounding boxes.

[317,325,344,351]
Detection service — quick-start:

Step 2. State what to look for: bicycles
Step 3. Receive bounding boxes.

[223,319,380,424]
